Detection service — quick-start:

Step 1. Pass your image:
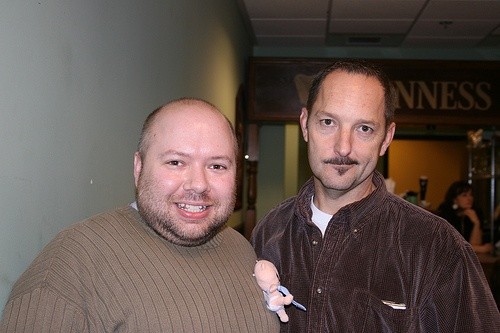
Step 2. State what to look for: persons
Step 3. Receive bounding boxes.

[433,180,496,254]
[1,97,284,332]
[249,59,500,332]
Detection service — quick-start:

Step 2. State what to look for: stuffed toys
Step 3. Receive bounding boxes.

[252,259,307,323]
